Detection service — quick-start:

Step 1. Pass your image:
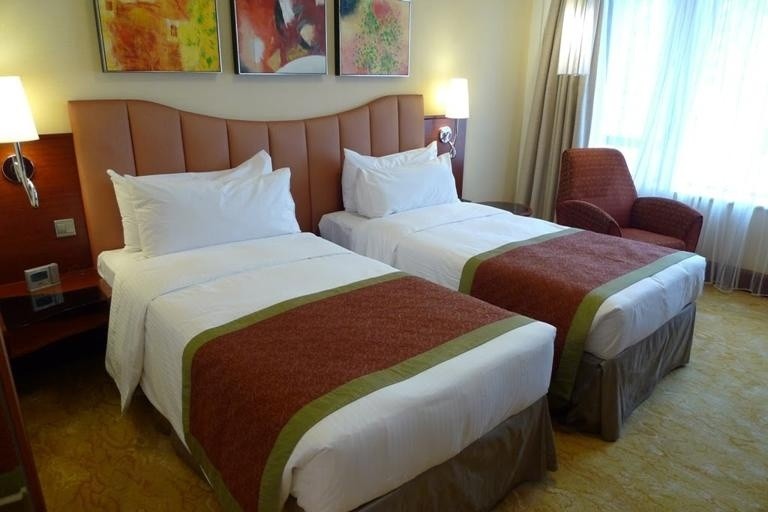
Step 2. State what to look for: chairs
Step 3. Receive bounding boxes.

[555,149,703,254]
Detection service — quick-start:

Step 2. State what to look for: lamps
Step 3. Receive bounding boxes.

[438,78,470,160]
[0,76,41,210]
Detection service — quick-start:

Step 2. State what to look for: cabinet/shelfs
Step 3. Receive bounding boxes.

[0,267,108,357]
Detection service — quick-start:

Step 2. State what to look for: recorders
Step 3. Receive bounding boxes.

[32,293,65,312]
[24,262,60,292]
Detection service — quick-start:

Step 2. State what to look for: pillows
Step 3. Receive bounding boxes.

[340,141,460,218]
[104,148,302,258]
